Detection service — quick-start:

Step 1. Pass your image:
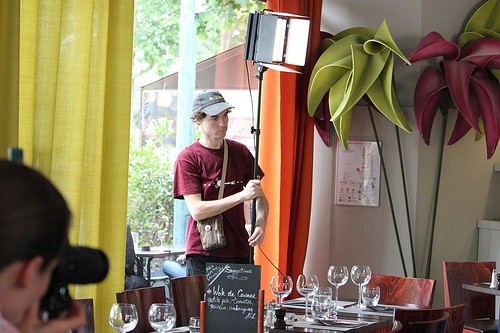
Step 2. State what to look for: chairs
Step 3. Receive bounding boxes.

[442,261,500,333]
[116,286,166,333]
[171,275,206,327]
[365,274,436,309]
[386,305,464,333]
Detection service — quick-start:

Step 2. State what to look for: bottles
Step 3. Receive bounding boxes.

[490,269,497,288]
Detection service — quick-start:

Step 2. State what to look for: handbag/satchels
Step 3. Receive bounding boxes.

[196,215,226,251]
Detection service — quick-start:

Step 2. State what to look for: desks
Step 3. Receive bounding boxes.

[461,282,500,321]
[152,296,395,333]
[133,249,187,286]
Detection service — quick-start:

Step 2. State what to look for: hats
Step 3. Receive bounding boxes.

[193,90,235,117]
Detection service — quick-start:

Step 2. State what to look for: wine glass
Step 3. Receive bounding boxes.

[296,274,319,322]
[270,275,293,307]
[351,265,371,310]
[158,231,165,248]
[327,264,349,309]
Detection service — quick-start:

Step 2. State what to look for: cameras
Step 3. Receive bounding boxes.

[38,242,108,320]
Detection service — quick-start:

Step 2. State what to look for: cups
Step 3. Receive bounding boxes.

[109,303,139,333]
[189,316,201,333]
[148,303,177,333]
[311,287,338,326]
[362,286,380,308]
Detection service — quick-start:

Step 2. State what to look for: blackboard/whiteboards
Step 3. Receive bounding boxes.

[200,263,264,333]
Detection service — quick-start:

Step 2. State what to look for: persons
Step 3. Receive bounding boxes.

[172,89,269,277]
[0,159,87,333]
